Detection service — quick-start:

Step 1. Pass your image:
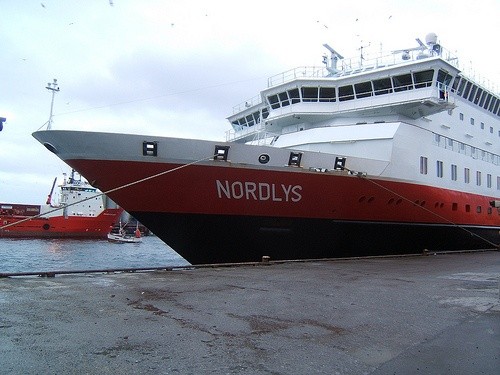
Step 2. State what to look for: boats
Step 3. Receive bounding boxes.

[25,12,499,269]
[0,168,125,240]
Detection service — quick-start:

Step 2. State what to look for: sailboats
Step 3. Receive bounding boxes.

[107,218,143,243]
